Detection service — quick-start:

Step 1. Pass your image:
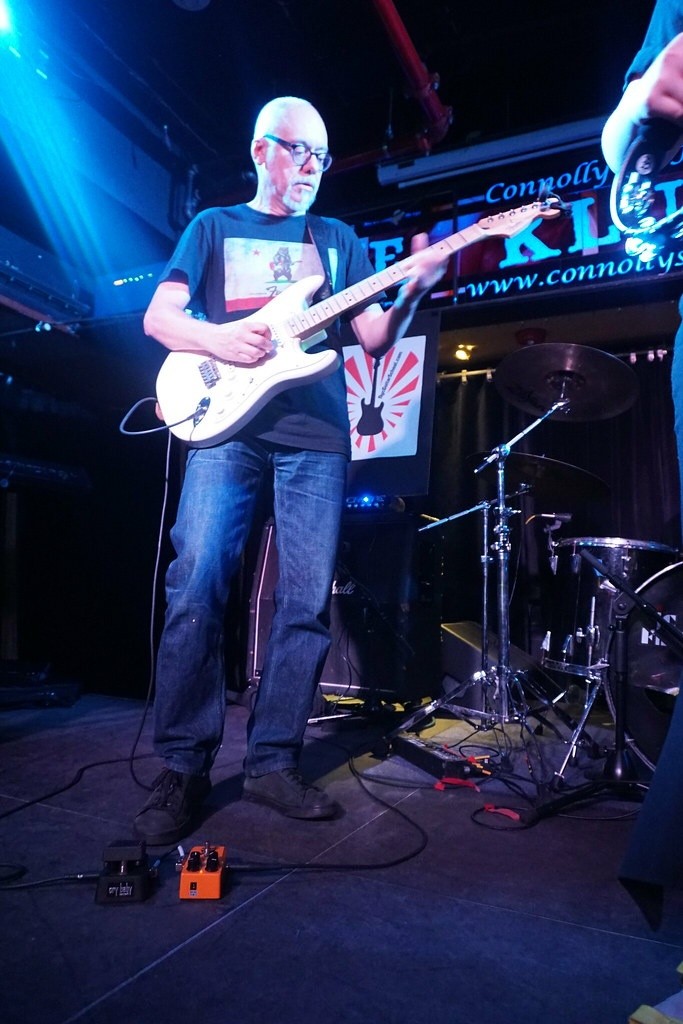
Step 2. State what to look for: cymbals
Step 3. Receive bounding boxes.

[471,449,614,509]
[493,342,639,424]
[608,113,683,265]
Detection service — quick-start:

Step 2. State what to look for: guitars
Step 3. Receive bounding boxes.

[153,197,562,451]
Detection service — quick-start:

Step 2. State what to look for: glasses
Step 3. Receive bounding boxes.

[263,135,332,171]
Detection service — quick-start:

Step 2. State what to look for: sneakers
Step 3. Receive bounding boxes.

[242,768,341,819]
[131,766,212,845]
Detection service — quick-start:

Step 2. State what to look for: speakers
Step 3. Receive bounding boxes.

[245,518,447,719]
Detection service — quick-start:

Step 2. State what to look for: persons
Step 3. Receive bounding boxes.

[602,0,683,466]
[136,96,449,847]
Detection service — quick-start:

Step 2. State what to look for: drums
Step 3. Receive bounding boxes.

[601,562,683,775]
[543,534,679,693]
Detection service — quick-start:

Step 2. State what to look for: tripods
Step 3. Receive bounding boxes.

[517,555,656,825]
[370,399,605,758]
